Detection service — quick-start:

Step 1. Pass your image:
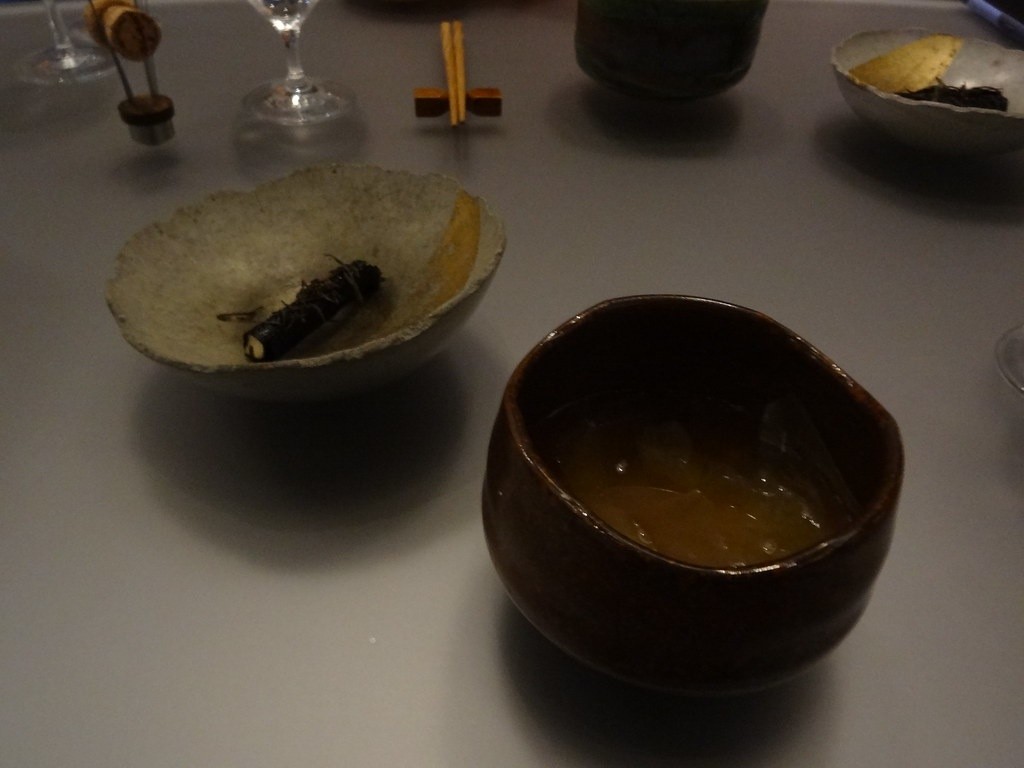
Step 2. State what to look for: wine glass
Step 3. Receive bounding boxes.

[9,0,124,82]
[242,0,353,124]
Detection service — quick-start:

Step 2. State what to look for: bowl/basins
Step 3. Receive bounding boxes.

[829,27,1024,163]
[483,295,904,705]
[100,166,506,394]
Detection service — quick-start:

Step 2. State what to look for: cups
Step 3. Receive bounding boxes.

[574,0,768,102]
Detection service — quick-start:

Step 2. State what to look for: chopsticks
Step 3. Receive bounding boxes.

[440,20,467,129]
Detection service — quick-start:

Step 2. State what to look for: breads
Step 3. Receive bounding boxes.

[850,34,961,93]
